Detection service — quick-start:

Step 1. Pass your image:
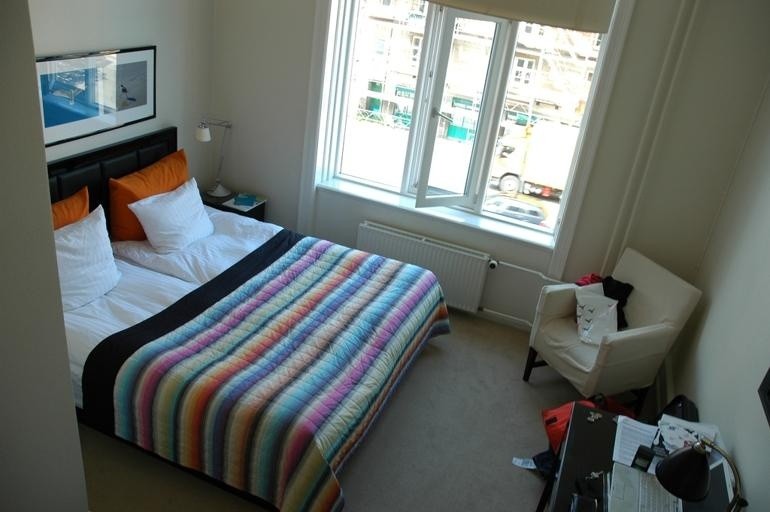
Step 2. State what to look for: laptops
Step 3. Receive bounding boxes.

[608,458,733,512]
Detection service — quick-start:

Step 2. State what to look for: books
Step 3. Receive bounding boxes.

[610,413,727,477]
[220,190,269,214]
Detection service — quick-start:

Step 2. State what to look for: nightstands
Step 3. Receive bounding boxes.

[200,191,266,222]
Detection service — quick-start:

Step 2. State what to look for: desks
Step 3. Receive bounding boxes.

[535,400,658,512]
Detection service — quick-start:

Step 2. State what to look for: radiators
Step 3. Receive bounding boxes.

[357,220,492,313]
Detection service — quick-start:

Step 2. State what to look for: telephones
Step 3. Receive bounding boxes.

[630,445,655,472]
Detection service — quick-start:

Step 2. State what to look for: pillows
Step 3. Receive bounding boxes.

[126,177,215,255]
[54,204,122,312]
[108,147,189,241]
[51,186,91,229]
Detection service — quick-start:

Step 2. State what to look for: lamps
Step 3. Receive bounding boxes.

[196,119,232,197]
[655,435,748,512]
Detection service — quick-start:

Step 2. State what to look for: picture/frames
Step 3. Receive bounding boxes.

[36,45,157,147]
[757,368,770,427]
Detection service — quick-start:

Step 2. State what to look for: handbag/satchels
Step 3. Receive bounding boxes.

[539,390,636,460]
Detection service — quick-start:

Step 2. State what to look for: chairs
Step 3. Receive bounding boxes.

[522,248,703,400]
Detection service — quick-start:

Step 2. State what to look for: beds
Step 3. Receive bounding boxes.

[46,125,451,511]
